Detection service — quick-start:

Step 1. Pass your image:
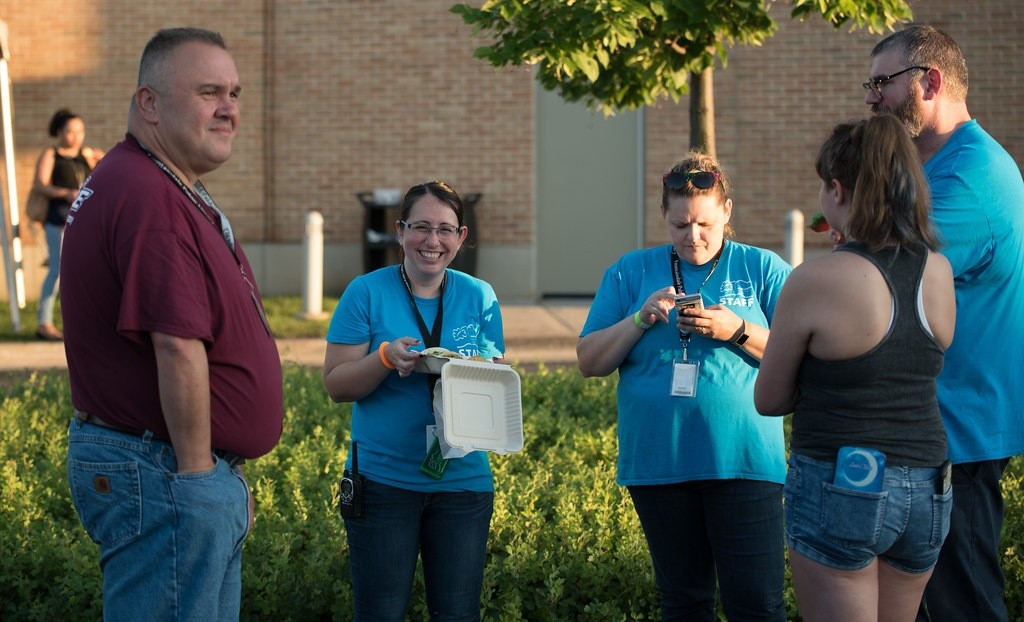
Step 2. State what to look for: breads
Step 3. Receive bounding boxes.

[467,355,487,361]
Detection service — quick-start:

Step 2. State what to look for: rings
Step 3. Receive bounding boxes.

[700,327,706,335]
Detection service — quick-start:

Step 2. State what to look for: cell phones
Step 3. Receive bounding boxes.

[833,447,886,494]
[674,293,705,319]
[938,460,952,495]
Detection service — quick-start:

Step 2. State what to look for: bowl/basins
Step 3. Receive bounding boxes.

[410,347,524,454]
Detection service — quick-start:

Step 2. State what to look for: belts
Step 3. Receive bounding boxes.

[74,409,245,465]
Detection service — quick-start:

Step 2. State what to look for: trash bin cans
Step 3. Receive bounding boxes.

[356,189,481,278]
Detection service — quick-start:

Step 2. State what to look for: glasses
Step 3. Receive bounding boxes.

[662,172,728,199]
[401,221,462,238]
[863,66,930,100]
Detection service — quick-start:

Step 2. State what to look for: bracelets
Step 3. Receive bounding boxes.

[634,310,653,329]
[378,341,395,369]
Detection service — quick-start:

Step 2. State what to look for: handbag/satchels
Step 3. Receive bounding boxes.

[26,146,58,221]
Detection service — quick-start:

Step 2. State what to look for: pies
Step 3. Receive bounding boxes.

[420,347,464,359]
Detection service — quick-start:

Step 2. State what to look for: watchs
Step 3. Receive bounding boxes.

[733,318,749,346]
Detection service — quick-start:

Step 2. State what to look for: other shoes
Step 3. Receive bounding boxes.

[35,327,64,340]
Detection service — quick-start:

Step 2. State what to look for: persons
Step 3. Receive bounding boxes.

[61,25,284,622]
[753,115,957,622]
[575,150,793,622]
[36,108,107,341]
[324,180,510,622]
[862,24,1024,622]
